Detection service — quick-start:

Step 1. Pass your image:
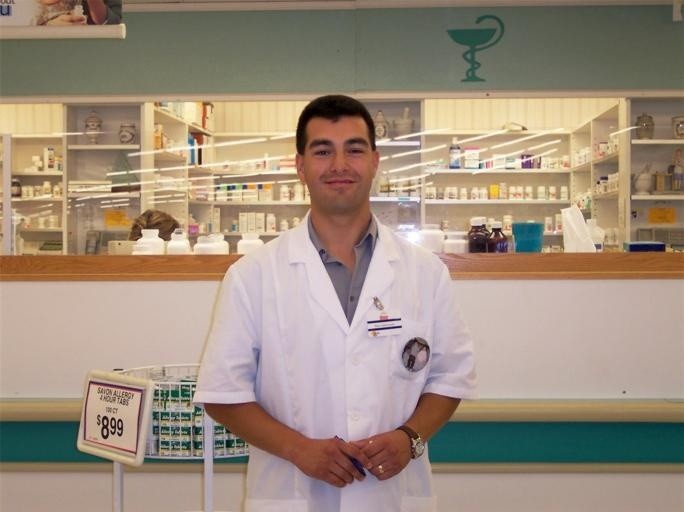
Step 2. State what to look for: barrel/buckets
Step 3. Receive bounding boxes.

[511,221,545,252]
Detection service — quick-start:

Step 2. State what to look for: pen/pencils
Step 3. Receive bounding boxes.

[335,436,366,477]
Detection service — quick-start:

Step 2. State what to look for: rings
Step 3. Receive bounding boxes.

[378,464,384,475]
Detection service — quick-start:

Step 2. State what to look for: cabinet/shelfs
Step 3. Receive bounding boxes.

[623,96,684,248]
[423,128,570,246]
[2,131,64,252]
[211,131,311,238]
[59,100,147,254]
[147,102,211,239]
[364,95,424,233]
[570,100,626,248]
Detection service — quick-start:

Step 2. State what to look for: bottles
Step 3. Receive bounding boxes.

[132,228,265,254]
[231,221,239,232]
[11,149,62,232]
[595,173,619,194]
[467,216,508,253]
[372,110,389,140]
[635,115,654,139]
[426,183,569,200]
[80,112,137,145]
[420,221,467,253]
[586,219,603,252]
[279,182,309,201]
[448,137,462,170]
[280,219,289,231]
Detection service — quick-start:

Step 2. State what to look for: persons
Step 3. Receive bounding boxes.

[193,95,481,512]
[39,0,123,25]
[129,209,183,242]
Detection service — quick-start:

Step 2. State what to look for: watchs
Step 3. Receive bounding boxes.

[396,425,425,460]
